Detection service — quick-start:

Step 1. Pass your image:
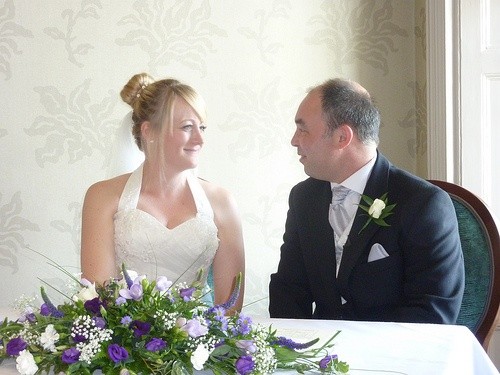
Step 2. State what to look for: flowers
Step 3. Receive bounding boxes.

[352,192,397,235]
[0,247,350,375]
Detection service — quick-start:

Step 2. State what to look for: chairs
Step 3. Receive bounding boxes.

[424,179,500,354]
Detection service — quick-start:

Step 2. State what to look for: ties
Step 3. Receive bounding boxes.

[328,185,351,238]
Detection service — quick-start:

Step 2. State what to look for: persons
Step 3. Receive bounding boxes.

[79,72,245,317]
[268,78,467,325]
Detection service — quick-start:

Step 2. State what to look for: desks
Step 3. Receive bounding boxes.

[0,318,500,374]
